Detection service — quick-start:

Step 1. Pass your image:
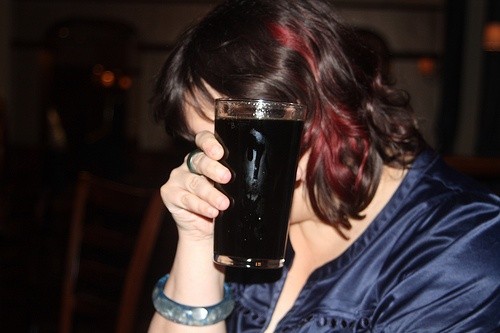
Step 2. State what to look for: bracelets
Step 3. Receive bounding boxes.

[152,275,235,326]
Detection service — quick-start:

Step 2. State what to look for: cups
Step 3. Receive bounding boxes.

[211,98,307,269]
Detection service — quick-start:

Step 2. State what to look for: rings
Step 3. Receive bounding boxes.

[187,152,206,176]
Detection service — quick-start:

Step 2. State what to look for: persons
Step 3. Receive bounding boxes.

[147,0,499,333]
